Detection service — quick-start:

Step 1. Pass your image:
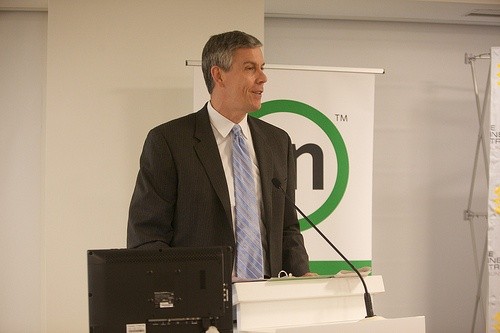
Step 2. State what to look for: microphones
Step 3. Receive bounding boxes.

[271,177,376,318]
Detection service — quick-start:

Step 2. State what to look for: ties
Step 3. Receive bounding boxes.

[231,124,263,280]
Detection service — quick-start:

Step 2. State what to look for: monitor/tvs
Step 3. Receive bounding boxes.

[86,245,234,333]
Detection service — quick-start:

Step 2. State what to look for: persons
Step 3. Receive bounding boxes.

[126,30,319,280]
